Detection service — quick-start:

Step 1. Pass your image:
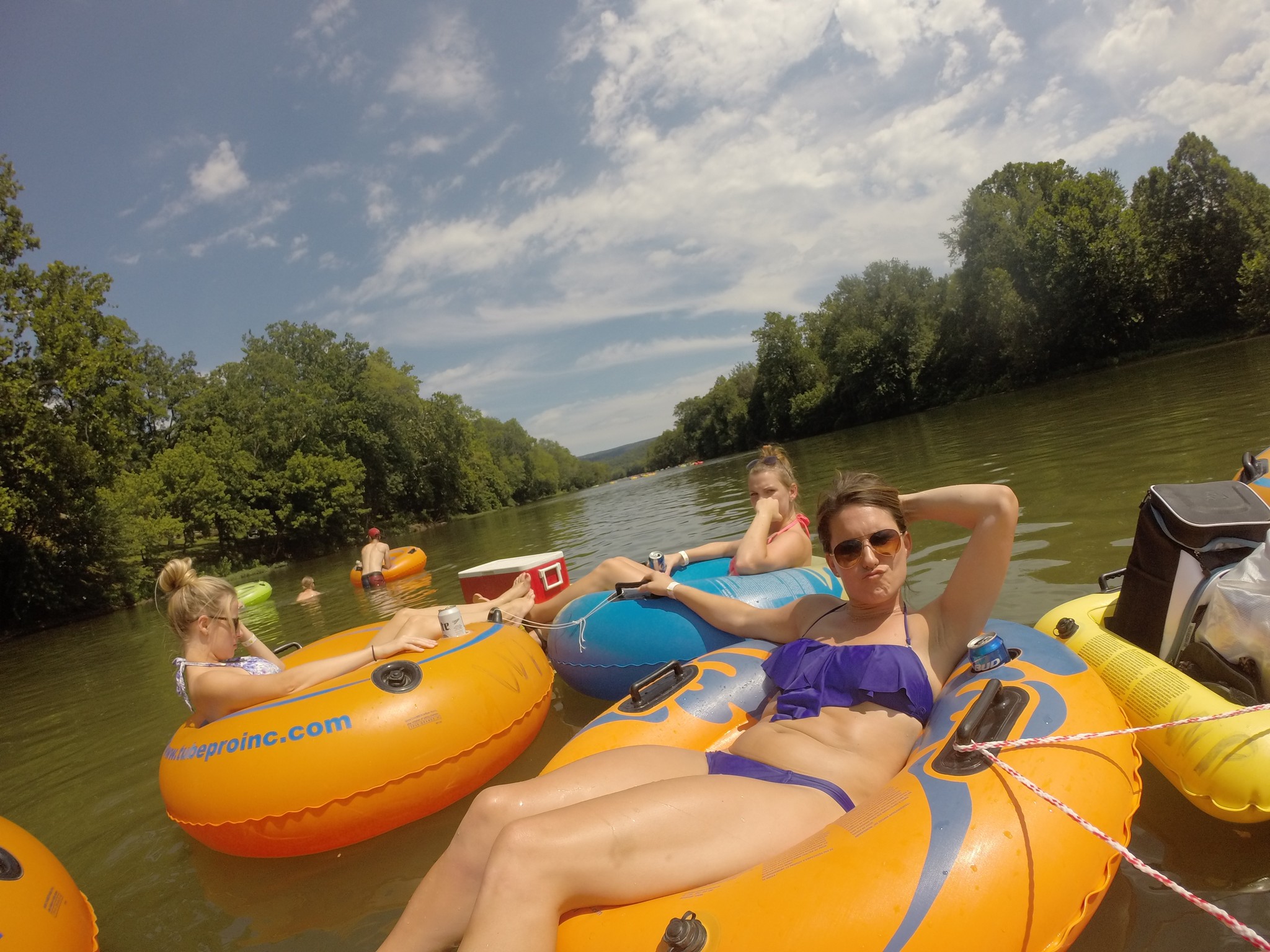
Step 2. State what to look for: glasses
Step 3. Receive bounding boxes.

[187,615,240,632]
[312,582,315,584]
[829,529,903,570]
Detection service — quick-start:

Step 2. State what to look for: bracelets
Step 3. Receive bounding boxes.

[372,645,377,661]
[678,551,689,566]
[666,582,681,601]
[240,632,257,647]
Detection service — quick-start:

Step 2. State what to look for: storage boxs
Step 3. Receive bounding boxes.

[457,551,570,606]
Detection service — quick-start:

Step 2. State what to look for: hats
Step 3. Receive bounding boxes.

[368,528,381,537]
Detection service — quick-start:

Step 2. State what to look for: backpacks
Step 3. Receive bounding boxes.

[1112,479,1270,665]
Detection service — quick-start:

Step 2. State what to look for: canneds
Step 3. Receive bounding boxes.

[356,560,360,566]
[649,550,666,572]
[966,631,1012,672]
[438,605,467,638]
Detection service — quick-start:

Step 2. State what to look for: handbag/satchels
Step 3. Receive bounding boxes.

[1193,529,1270,696]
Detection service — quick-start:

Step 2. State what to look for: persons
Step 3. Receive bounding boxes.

[472,444,812,651]
[296,576,320,601]
[160,557,535,723]
[371,468,1020,952]
[361,527,391,589]
